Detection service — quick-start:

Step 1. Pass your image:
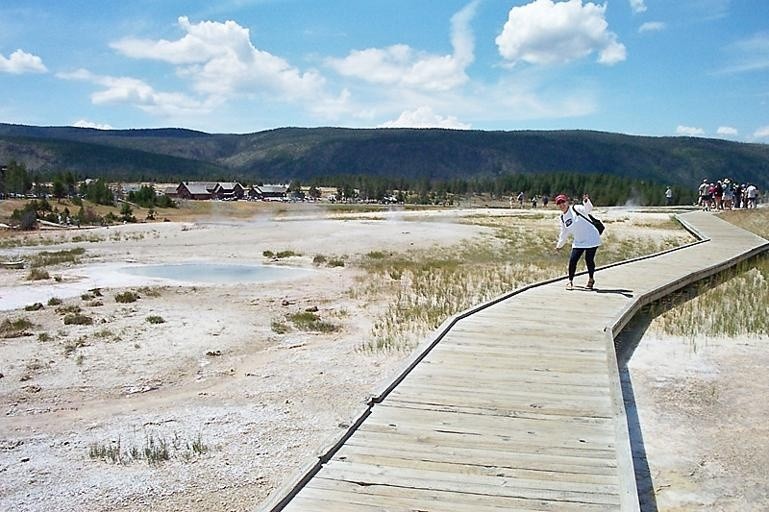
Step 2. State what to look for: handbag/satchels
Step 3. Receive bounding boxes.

[592,219,604,235]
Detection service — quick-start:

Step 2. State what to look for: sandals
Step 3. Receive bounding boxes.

[585,280,594,289]
[566,282,574,290]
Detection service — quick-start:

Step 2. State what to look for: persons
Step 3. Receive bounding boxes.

[553,193,603,291]
[509,192,588,209]
[698,175,761,212]
[664,186,673,206]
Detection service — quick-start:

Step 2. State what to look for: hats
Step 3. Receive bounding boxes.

[724,179,730,183]
[555,194,568,205]
